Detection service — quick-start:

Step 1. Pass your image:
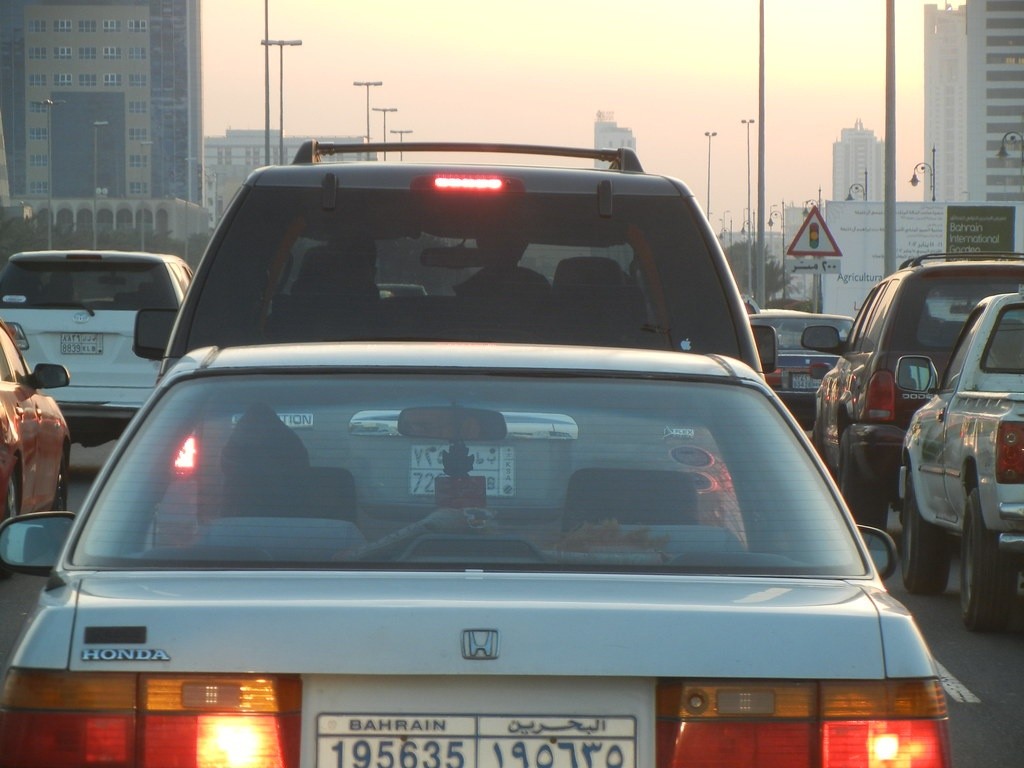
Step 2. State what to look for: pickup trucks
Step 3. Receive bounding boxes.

[892,293,1024,637]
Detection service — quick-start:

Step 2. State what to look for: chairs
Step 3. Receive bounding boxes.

[548,255,649,331]
[289,249,387,347]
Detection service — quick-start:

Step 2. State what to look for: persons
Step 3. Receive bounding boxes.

[917,301,938,342]
[272,228,382,324]
[202,401,336,519]
[46,270,75,304]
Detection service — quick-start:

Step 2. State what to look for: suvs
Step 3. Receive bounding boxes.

[798,254,1024,532]
[0,248,195,449]
[133,137,781,548]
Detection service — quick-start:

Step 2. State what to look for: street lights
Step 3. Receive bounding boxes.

[801,187,822,218]
[390,130,414,162]
[371,107,399,162]
[723,210,731,251]
[29,98,66,249]
[844,167,868,201]
[740,118,756,297]
[703,130,718,221]
[767,200,787,309]
[740,211,757,287]
[994,129,1024,203]
[92,120,109,252]
[353,80,384,160]
[718,219,733,270]
[908,144,937,202]
[260,39,303,165]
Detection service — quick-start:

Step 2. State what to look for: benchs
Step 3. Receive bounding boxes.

[187,516,747,572]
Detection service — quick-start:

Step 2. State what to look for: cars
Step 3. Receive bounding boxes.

[0,339,953,768]
[743,293,856,431]
[0,314,75,584]
